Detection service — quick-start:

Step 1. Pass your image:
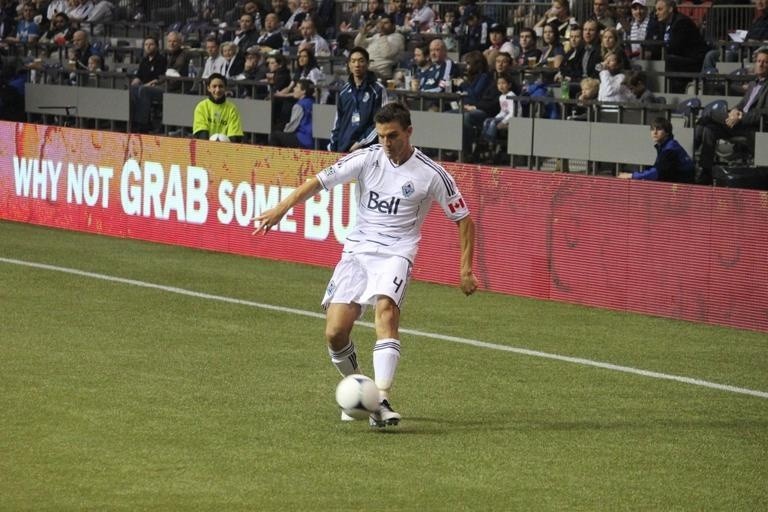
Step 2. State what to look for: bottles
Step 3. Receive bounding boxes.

[358,15,365,31]
[561,76,570,100]
[280,36,293,55]
[254,11,261,30]
[304,14,310,22]
[187,58,195,78]
[446,72,453,92]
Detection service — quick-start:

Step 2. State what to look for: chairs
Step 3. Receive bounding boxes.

[0,0,768,169]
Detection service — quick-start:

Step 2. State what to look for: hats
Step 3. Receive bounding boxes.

[630,0,647,8]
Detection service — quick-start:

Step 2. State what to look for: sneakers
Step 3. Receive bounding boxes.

[368,397,402,429]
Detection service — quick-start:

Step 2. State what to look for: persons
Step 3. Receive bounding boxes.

[693,46,768,184]
[701,0,768,74]
[248,104,479,425]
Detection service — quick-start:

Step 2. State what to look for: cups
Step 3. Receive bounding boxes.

[435,20,443,34]
[387,78,395,89]
[526,56,537,67]
[402,72,421,91]
[266,70,274,84]
[413,20,421,33]
[330,41,339,56]
[68,58,76,69]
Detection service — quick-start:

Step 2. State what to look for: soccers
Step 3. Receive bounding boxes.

[336,374,380,419]
[209,133,231,142]
